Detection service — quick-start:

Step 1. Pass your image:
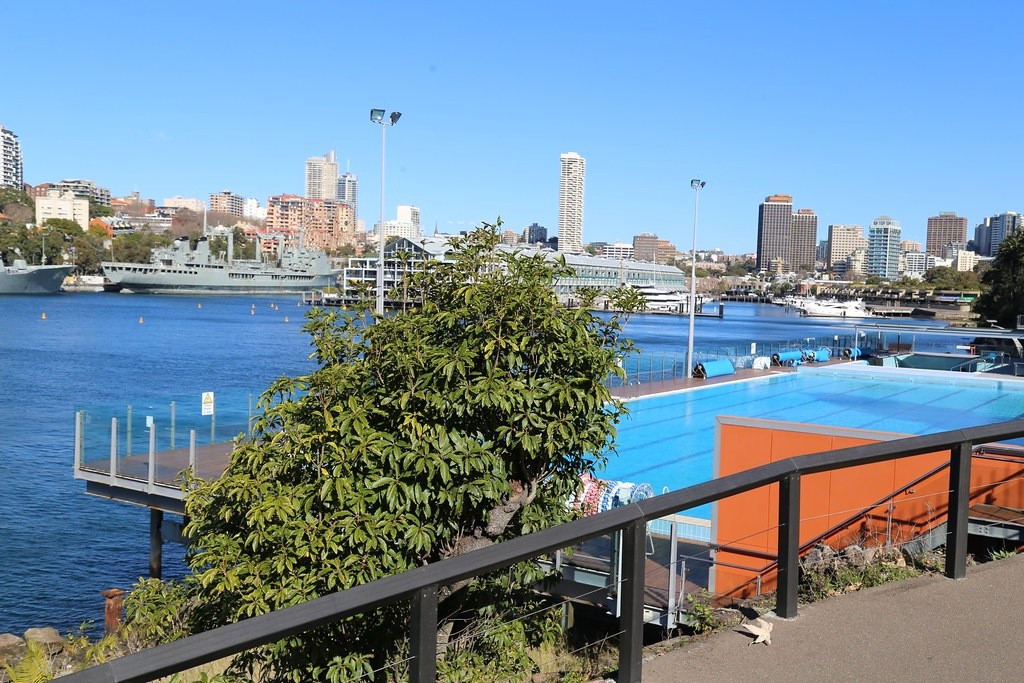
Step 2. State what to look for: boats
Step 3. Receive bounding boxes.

[796,297,877,318]
[0,237,78,296]
[631,283,684,310]
[100,231,344,293]
[966,336,1024,358]
[61,275,123,292]
[773,295,796,306]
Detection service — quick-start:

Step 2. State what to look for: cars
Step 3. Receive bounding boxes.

[763,292,774,296]
[785,296,793,301]
[748,293,758,297]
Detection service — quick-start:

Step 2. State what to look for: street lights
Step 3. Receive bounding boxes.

[370,108,402,324]
[687,179,708,378]
